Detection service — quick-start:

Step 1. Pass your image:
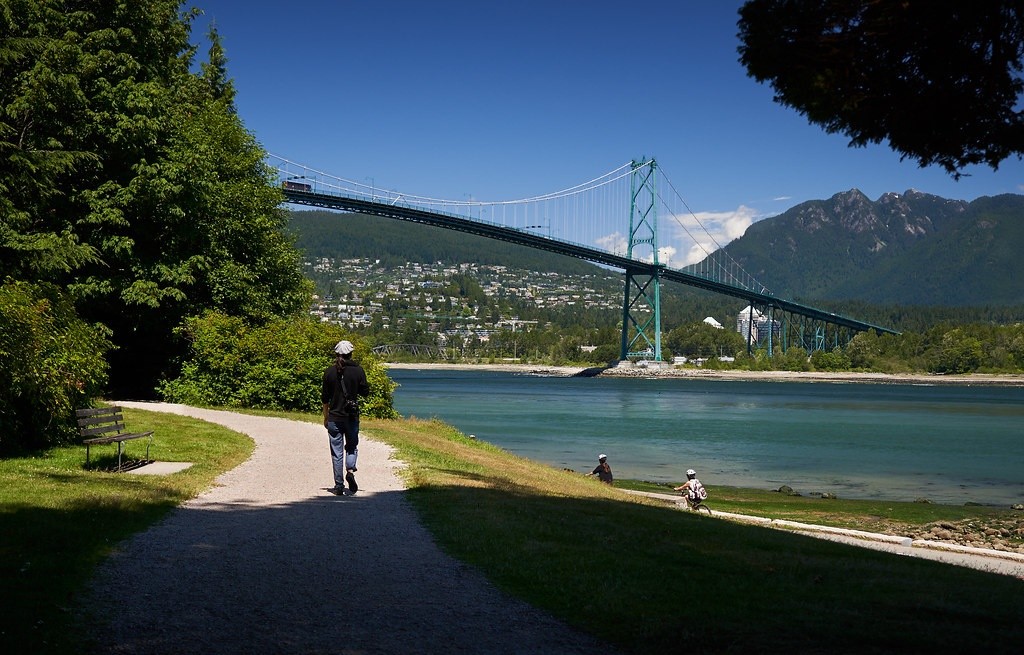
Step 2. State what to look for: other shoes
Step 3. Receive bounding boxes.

[346,472,358,492]
[331,488,344,495]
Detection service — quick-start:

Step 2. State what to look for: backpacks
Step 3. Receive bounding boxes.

[689,481,708,501]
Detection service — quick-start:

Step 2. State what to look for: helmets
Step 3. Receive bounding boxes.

[686,468,696,475]
[334,340,355,354]
[598,454,607,460]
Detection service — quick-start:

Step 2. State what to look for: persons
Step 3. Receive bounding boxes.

[673,469,701,512]
[322,340,370,494]
[585,455,612,484]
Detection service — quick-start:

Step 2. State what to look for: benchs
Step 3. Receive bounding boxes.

[75,406,154,472]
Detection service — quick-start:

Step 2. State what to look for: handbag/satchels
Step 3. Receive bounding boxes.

[344,398,359,414]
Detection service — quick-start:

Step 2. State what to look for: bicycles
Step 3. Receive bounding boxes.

[673,488,711,515]
